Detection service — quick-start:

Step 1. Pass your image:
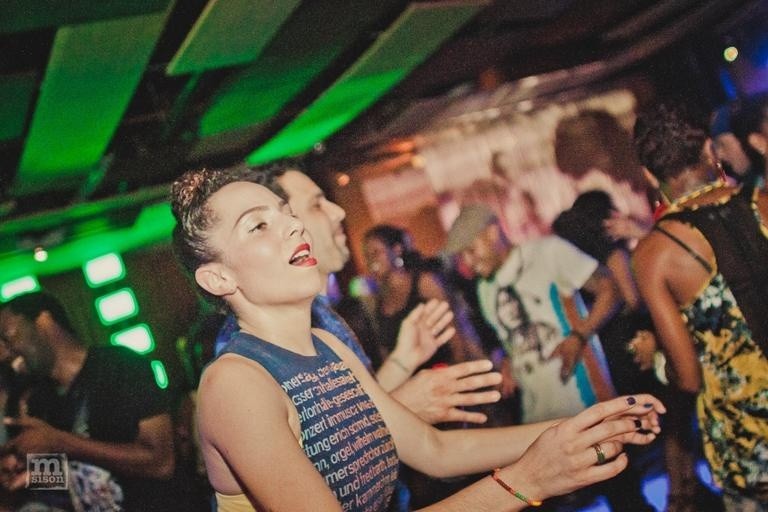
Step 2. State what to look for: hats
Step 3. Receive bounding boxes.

[444,205,494,259]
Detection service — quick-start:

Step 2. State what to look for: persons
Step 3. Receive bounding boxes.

[169,168,666,512]
[212,158,503,512]
[338,96,767,512]
[0,292,207,511]
